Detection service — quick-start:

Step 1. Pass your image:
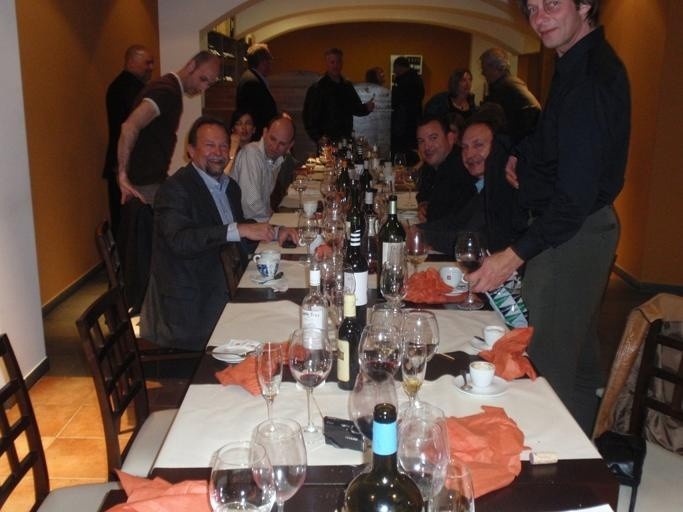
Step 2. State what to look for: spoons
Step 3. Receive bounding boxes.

[253,273,285,287]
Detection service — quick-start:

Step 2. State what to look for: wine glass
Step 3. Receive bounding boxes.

[208,342,308,512]
[289,133,353,437]
[408,227,431,276]
[454,232,485,311]
[401,166,419,208]
[352,304,478,512]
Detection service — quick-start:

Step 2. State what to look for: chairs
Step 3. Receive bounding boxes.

[591,291,683,512]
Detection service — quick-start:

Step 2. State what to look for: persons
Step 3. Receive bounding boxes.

[99,0,630,439]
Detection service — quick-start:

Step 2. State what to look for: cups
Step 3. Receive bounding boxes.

[468,358,495,389]
[439,265,469,287]
[254,249,282,277]
[485,327,507,348]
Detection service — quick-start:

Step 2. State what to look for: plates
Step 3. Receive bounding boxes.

[471,337,492,354]
[454,372,509,396]
[398,211,421,218]
[441,287,470,296]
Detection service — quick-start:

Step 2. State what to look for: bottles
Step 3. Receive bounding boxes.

[392,57,420,87]
[337,128,407,391]
[336,403,427,512]
